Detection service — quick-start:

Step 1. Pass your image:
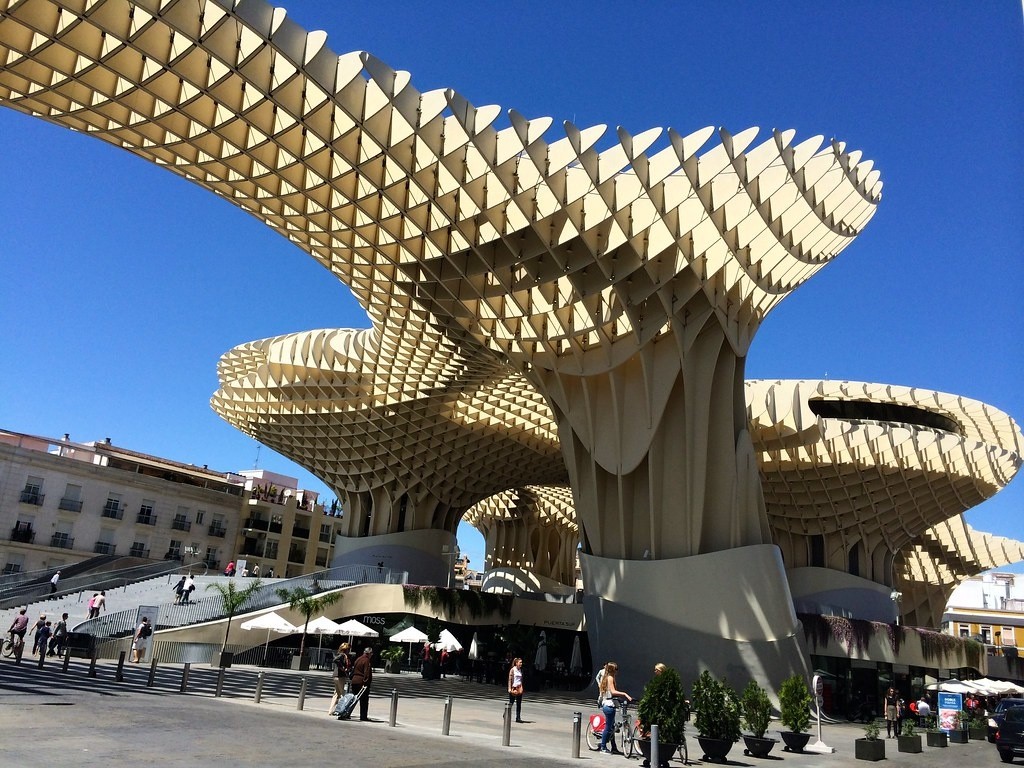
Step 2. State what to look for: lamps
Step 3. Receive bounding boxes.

[643,550,651,559]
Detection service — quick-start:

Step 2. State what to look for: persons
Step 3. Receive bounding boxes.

[8,561,276,664]
[329,642,509,720]
[884,687,979,740]
[507,657,525,723]
[596,662,666,754]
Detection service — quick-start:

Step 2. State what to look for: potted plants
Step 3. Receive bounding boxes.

[688,669,742,763]
[336,506,344,518]
[741,679,780,758]
[379,645,405,674]
[636,666,690,768]
[777,671,814,752]
[949,711,970,743]
[854,719,886,761]
[251,482,286,505]
[323,510,328,515]
[968,718,988,740]
[923,713,948,748]
[897,718,923,753]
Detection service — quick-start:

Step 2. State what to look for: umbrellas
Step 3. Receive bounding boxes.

[535,631,548,671]
[927,678,1024,698]
[389,627,478,665]
[241,611,379,660]
[570,636,584,676]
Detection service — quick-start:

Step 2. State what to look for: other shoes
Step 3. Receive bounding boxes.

[443,676,446,678]
[886,736,898,740]
[600,747,613,755]
[359,718,372,721]
[611,748,624,755]
[516,719,523,723]
[598,703,601,708]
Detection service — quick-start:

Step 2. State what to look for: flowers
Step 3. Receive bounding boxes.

[796,611,1020,679]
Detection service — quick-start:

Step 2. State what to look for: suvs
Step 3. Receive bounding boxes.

[996,704,1024,762]
[987,696,1024,743]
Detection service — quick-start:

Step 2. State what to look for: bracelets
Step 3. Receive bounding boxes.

[885,711,886,712]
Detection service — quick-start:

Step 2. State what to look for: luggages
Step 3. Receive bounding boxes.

[333,685,367,720]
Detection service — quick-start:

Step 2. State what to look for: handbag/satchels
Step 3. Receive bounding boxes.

[350,669,355,680]
[338,655,349,677]
[511,684,523,696]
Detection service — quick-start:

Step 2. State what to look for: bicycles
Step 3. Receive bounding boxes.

[1,631,20,658]
[586,697,639,758]
[632,703,691,765]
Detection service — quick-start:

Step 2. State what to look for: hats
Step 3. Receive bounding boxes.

[424,640,432,646]
[656,663,666,672]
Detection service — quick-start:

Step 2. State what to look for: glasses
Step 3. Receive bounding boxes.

[891,691,894,692]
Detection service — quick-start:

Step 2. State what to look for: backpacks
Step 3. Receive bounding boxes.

[140,622,152,638]
[420,647,426,658]
